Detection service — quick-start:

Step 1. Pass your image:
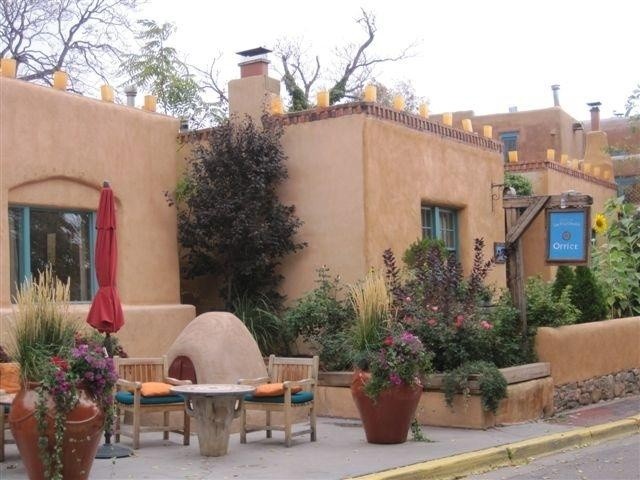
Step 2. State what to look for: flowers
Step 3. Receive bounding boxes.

[335,269,439,443]
[0,262,129,480]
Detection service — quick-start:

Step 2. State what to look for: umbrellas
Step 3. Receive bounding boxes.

[79,178,135,360]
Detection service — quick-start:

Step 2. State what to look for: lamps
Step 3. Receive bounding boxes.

[492,182,516,200]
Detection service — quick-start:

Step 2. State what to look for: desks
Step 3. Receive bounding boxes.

[169,383,257,457]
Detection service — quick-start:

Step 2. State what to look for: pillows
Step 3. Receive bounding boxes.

[253,382,303,397]
[141,381,177,398]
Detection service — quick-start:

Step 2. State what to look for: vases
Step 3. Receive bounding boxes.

[8,381,105,480]
[350,369,424,444]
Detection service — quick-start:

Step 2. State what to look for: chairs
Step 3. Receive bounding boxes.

[0,361,41,461]
[237,354,319,448]
[113,354,191,450]
